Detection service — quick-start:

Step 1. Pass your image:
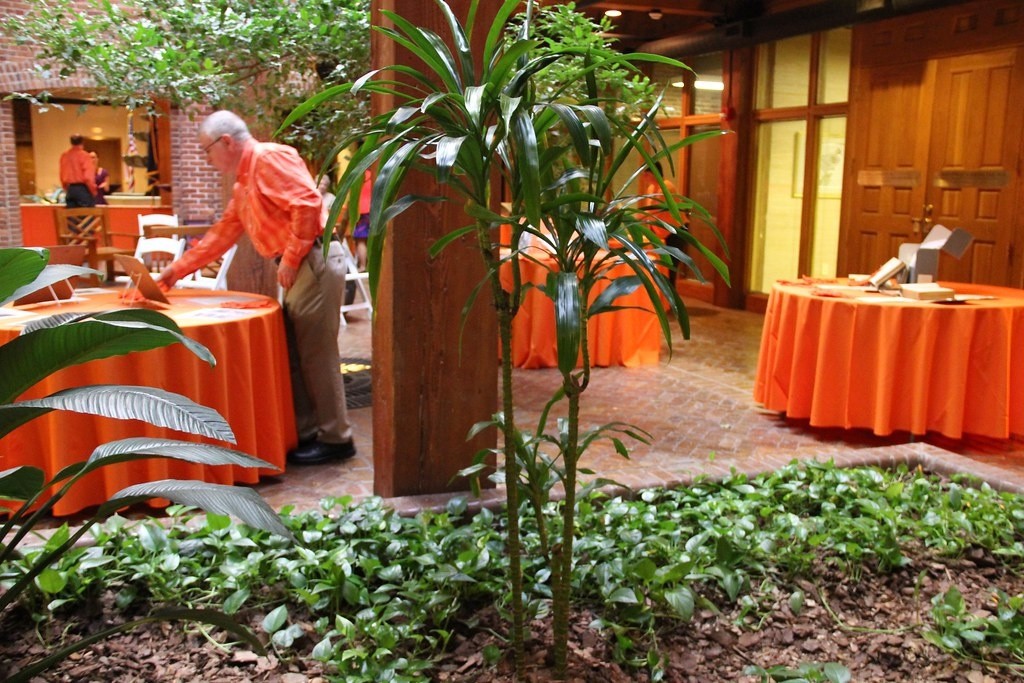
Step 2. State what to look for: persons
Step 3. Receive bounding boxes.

[152,109,357,466]
[90,151,110,205]
[632,159,691,340]
[59,133,97,237]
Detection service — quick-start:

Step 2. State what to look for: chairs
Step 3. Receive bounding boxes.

[321,236,372,325]
[128,212,238,291]
[55,207,134,289]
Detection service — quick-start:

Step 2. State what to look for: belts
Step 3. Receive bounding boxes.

[69,184,86,187]
[274,235,339,266]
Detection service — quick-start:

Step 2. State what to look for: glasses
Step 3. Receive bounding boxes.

[202,135,223,155]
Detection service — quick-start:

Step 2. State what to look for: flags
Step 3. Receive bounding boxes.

[126,118,137,193]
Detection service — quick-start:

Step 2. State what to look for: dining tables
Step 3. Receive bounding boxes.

[754,278,1024,442]
[0,286,297,515]
[498,201,670,367]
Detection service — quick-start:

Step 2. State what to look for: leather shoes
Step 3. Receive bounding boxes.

[291,434,317,447]
[286,435,356,465]
